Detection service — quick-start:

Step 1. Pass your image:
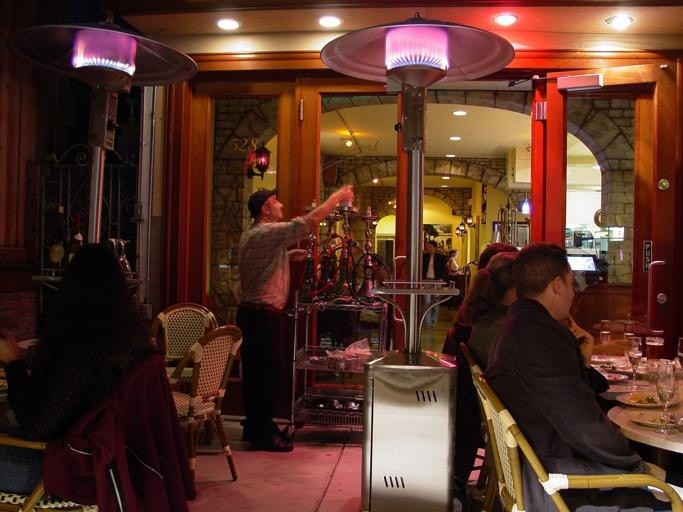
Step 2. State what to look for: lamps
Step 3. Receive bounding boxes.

[454,213,476,239]
[521,188,530,215]
[245,144,271,182]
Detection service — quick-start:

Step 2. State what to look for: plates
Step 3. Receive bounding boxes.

[590,358,614,365]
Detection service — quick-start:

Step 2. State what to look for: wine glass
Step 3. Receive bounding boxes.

[598,329,683,435]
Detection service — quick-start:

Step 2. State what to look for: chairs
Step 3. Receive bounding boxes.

[0,284,242,512]
[440,320,682,511]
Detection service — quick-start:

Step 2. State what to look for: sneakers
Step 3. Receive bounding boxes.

[425,320,439,330]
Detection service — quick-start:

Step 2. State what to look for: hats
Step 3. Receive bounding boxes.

[247,189,279,216]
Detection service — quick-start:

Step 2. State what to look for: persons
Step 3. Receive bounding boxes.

[445,250,460,310]
[238,182,354,451]
[423,240,449,329]
[484,243,683,511]
[453,242,519,343]
[0,243,155,495]
[456,252,517,370]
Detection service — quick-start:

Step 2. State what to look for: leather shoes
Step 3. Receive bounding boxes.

[242,425,295,451]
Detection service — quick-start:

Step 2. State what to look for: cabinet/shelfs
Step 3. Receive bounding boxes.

[286,292,389,437]
